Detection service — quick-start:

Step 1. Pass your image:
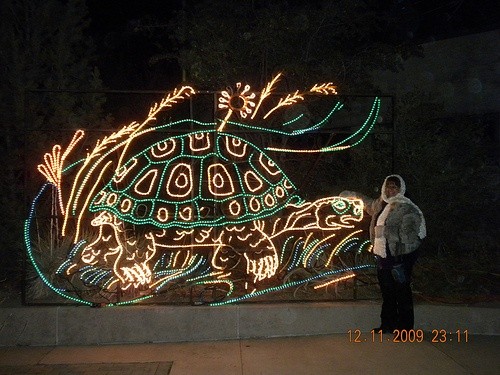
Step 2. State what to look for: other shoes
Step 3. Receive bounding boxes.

[373,326,394,334]
[392,333,415,343]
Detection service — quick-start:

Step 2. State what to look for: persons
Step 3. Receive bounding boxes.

[337,173,429,341]
